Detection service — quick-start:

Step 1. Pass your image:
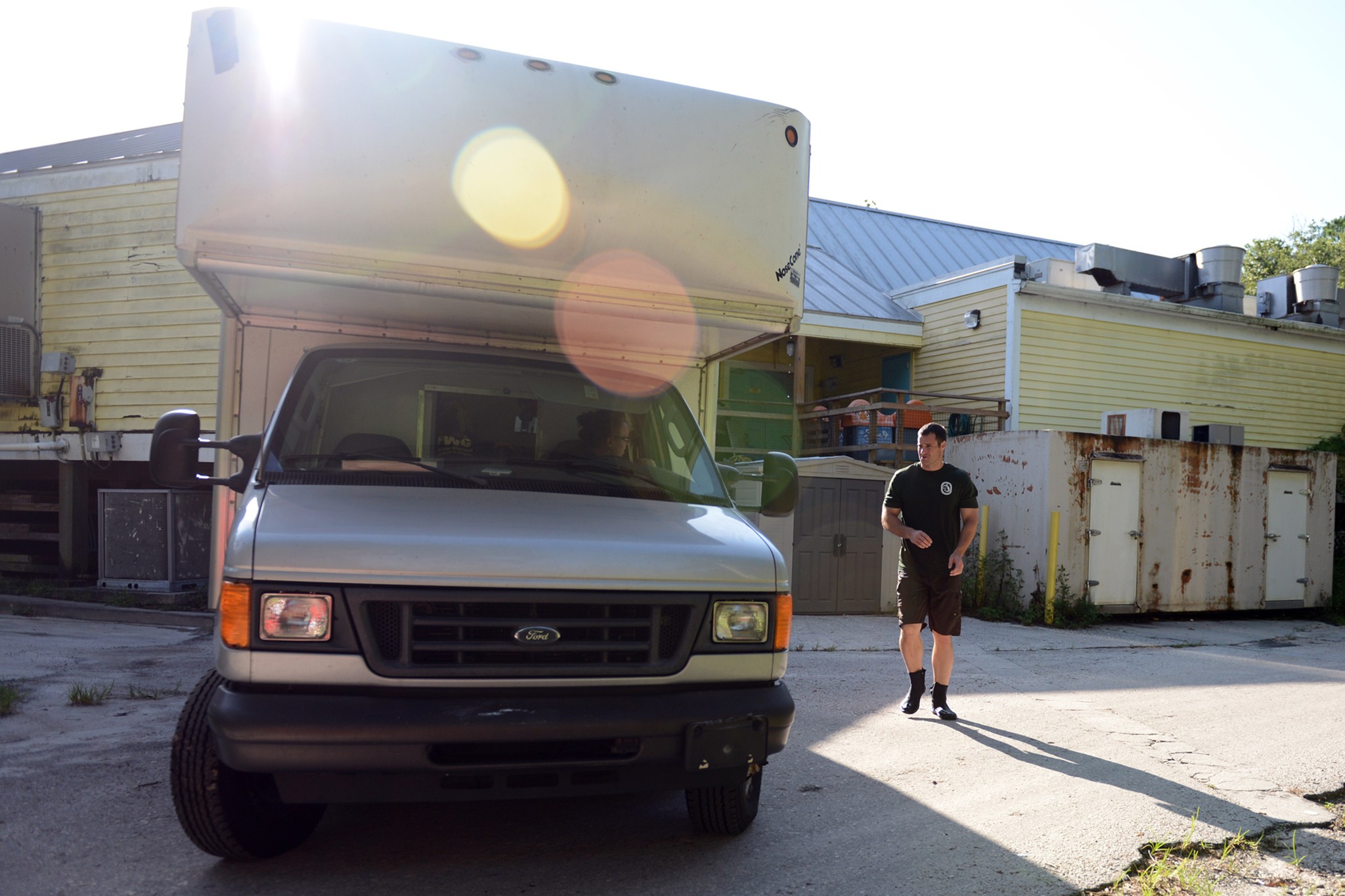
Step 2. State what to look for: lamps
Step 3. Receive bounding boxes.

[965,310,980,329]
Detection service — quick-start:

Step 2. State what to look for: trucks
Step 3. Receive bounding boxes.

[150,3,813,859]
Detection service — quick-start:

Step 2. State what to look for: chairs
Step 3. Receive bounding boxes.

[324,434,413,467]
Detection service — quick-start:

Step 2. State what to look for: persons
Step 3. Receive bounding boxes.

[544,410,656,470]
[881,421,980,719]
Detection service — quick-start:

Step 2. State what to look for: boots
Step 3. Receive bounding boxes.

[929,682,957,721]
[900,667,926,714]
